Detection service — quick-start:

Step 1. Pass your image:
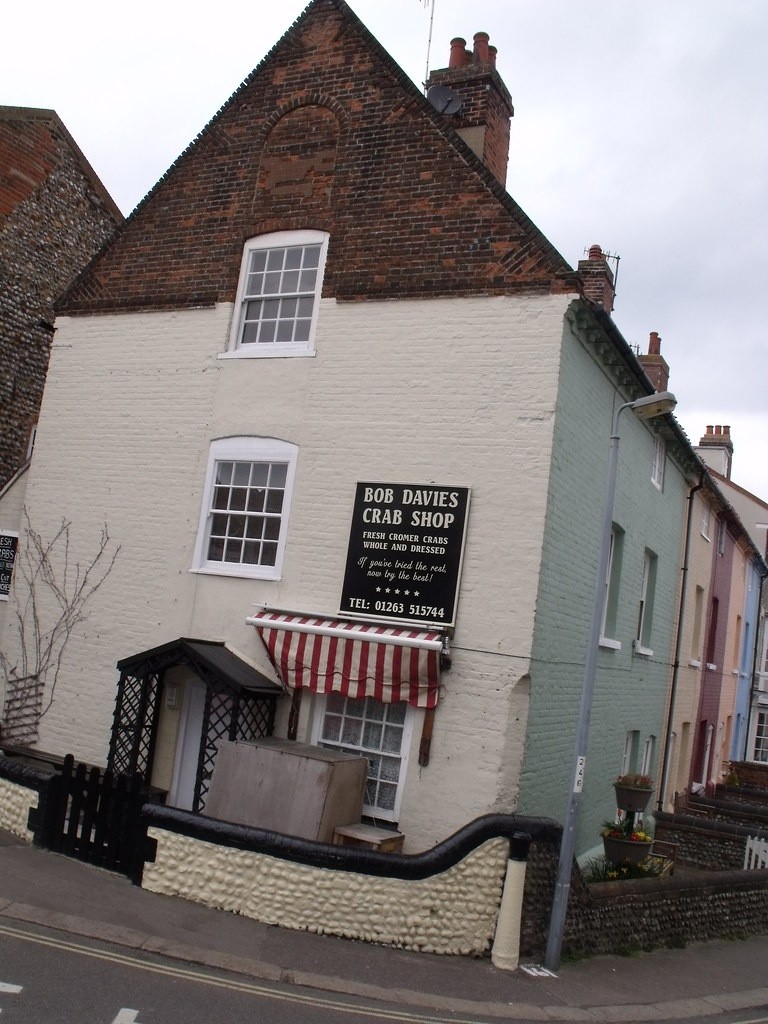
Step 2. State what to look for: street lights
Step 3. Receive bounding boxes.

[542,391,681,974]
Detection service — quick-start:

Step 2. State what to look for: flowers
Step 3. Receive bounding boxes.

[617,773,653,789]
[600,817,653,842]
[581,855,673,884]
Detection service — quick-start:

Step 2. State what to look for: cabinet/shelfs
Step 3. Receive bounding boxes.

[332,824,405,854]
[202,737,368,844]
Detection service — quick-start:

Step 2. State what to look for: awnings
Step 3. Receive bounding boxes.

[246,602,453,708]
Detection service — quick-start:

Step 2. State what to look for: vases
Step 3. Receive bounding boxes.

[600,834,655,864]
[613,784,656,812]
